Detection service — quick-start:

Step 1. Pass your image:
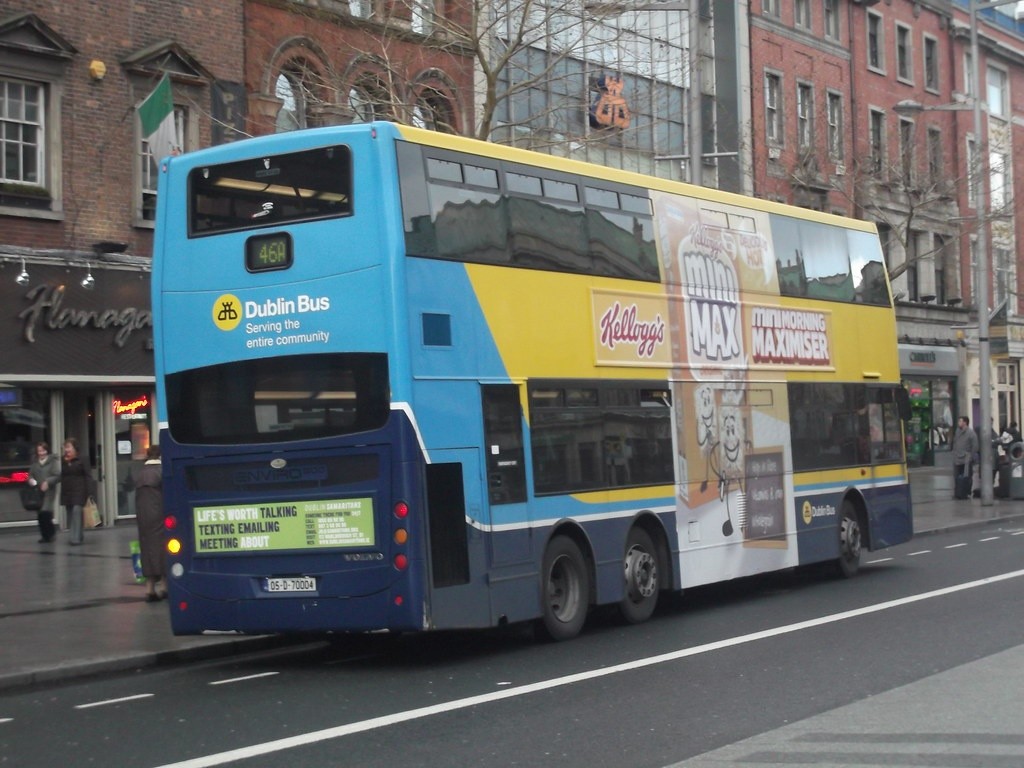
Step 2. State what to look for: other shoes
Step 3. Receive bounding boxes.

[159,591,169,600]
[38,537,55,545]
[144,592,162,602]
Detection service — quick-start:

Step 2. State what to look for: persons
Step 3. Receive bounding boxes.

[134,445,165,601]
[952,416,1023,498]
[60,437,96,547]
[25,442,60,543]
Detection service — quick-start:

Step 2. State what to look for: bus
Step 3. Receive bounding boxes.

[151,120,915,645]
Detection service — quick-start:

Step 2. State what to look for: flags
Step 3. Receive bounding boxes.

[136,74,180,166]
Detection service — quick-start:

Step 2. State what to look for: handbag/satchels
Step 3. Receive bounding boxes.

[19,478,45,510]
[82,496,102,528]
[129,540,149,584]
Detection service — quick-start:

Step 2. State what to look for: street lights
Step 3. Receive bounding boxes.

[891,1,1023,508]
